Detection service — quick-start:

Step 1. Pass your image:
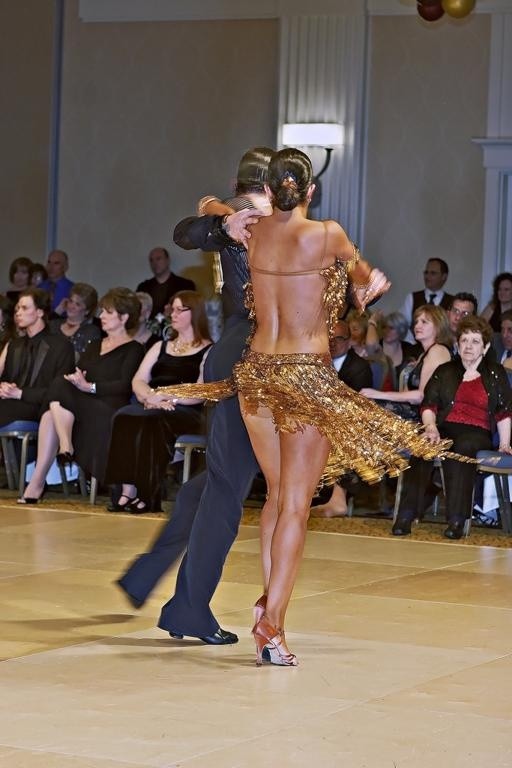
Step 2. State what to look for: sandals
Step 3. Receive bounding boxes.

[123,497,150,514]
[310,503,347,519]
[107,494,136,512]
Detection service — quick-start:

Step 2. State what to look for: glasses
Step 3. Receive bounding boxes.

[424,270,443,277]
[171,307,191,313]
[330,336,351,343]
[451,307,471,317]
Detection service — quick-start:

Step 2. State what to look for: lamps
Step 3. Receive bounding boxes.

[279,120,347,210]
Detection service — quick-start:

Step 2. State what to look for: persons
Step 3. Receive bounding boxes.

[152,147,501,668]
[113,145,391,646]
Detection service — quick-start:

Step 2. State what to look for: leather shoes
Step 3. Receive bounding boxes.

[168,624,240,646]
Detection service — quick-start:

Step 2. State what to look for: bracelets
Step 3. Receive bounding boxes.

[198,194,219,215]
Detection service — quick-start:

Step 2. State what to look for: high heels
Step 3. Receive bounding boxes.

[251,618,300,666]
[16,479,48,505]
[56,449,77,477]
[253,595,271,659]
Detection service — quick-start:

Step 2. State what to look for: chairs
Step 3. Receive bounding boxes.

[174,433,208,483]
[0,419,98,505]
[347,430,511,539]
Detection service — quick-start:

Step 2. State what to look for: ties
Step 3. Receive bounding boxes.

[428,294,438,306]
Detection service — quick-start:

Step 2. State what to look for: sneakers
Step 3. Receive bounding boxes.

[391,515,412,536]
[444,521,464,539]
